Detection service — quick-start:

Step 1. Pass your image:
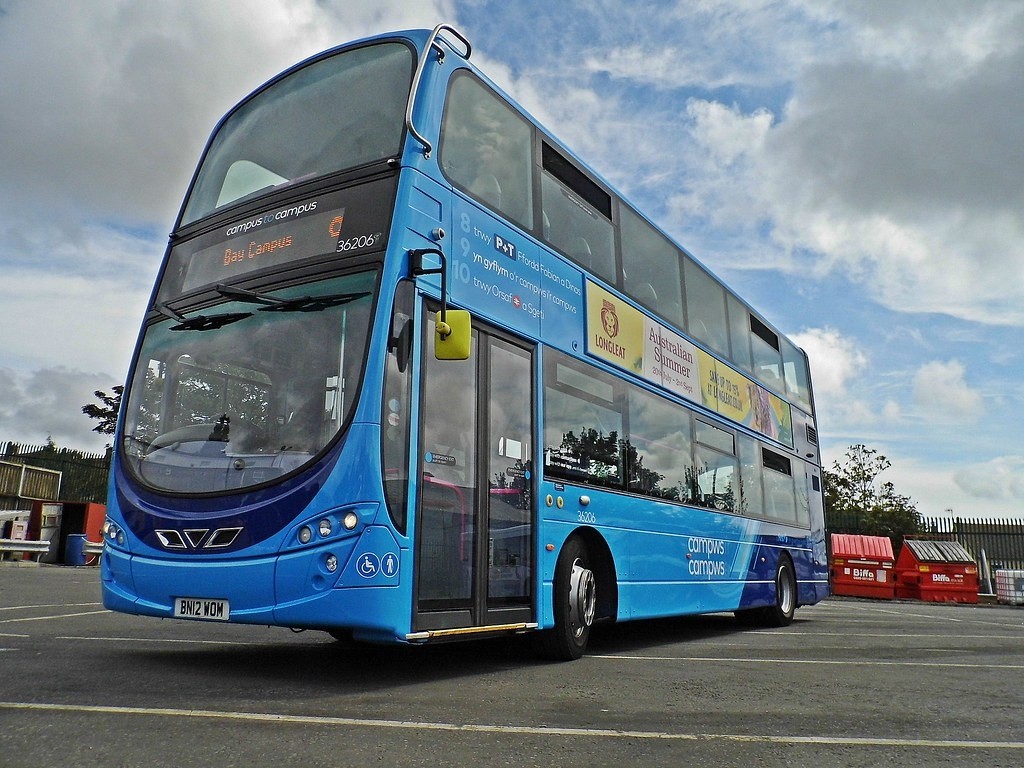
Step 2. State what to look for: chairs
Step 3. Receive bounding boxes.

[570,237,591,268]
[773,490,794,520]
[640,283,658,311]
[470,174,502,208]
[691,318,708,337]
[521,206,550,240]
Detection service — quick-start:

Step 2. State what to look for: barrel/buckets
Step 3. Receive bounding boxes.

[64,534,87,565]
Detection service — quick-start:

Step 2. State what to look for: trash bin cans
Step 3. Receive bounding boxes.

[64,534,87,566]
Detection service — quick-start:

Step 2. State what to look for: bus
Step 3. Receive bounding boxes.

[97,22,832,664]
